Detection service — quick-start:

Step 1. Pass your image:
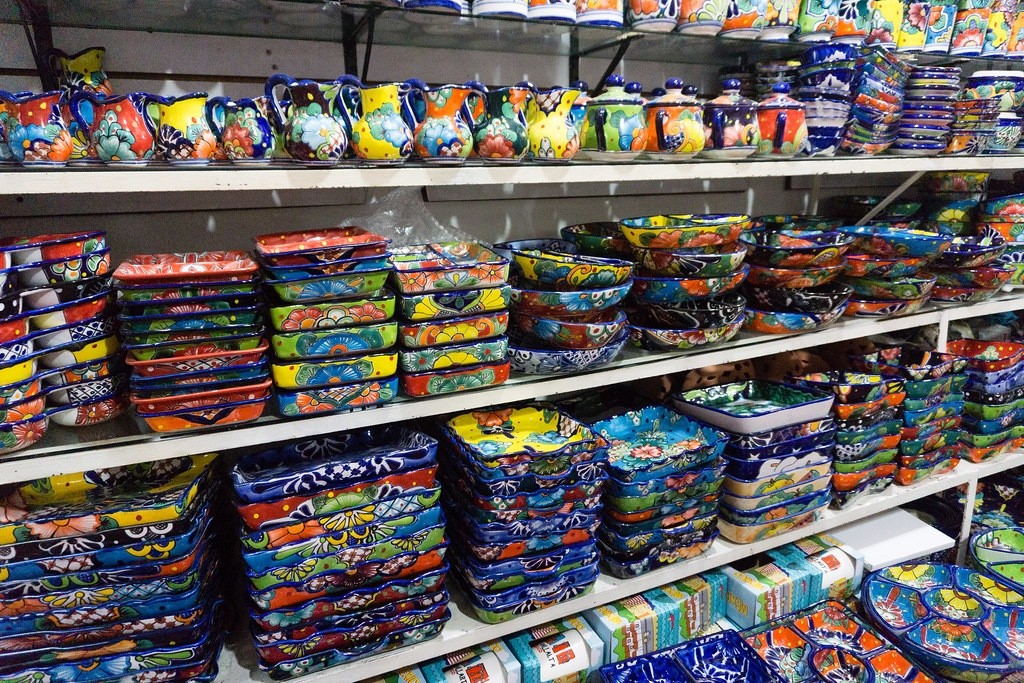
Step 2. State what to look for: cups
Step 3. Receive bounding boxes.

[645,80,705,159]
[579,75,648,161]
[757,83,808,158]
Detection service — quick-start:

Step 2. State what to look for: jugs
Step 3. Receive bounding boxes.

[405,79,472,165]
[0,90,73,168]
[0,92,33,163]
[335,74,413,165]
[463,81,530,163]
[69,91,155,166]
[142,93,216,166]
[208,95,276,166]
[44,46,114,99]
[264,73,348,167]
[516,82,581,163]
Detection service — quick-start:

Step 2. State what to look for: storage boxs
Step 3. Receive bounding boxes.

[421,637,521,683]
[507,613,604,683]
[659,569,728,641]
[583,589,682,665]
[776,532,865,605]
[717,549,812,630]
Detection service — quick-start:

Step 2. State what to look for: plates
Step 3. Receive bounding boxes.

[0,173,1024,683]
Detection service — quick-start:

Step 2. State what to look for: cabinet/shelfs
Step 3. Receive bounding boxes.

[0,0,1024,683]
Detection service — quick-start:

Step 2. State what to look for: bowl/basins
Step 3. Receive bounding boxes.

[719,42,1024,156]
